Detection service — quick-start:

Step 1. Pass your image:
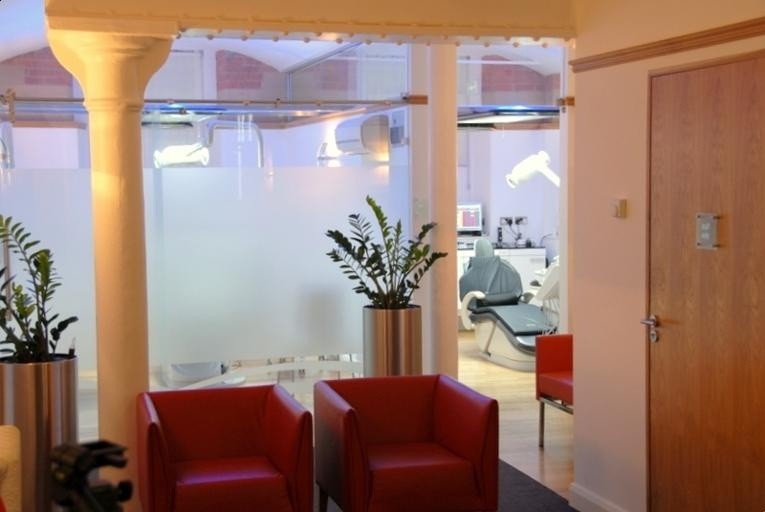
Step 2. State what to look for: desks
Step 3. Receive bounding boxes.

[456,248,548,332]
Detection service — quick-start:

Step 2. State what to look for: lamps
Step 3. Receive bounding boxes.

[456,109,561,125]
[141,110,217,171]
[317,138,343,168]
[501,149,560,192]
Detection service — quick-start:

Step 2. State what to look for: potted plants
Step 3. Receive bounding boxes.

[0,212,81,512]
[325,194,448,377]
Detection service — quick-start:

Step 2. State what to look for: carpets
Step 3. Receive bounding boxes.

[313,452,582,511]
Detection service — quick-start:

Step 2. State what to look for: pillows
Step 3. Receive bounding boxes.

[475,292,520,309]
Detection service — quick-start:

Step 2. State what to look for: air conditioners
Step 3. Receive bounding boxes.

[333,114,390,156]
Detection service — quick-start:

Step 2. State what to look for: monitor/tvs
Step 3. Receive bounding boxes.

[457,203,482,236]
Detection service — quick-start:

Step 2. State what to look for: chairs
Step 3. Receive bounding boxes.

[312,373,501,511]
[472,237,495,257]
[134,380,312,512]
[458,255,561,372]
[534,331,573,449]
